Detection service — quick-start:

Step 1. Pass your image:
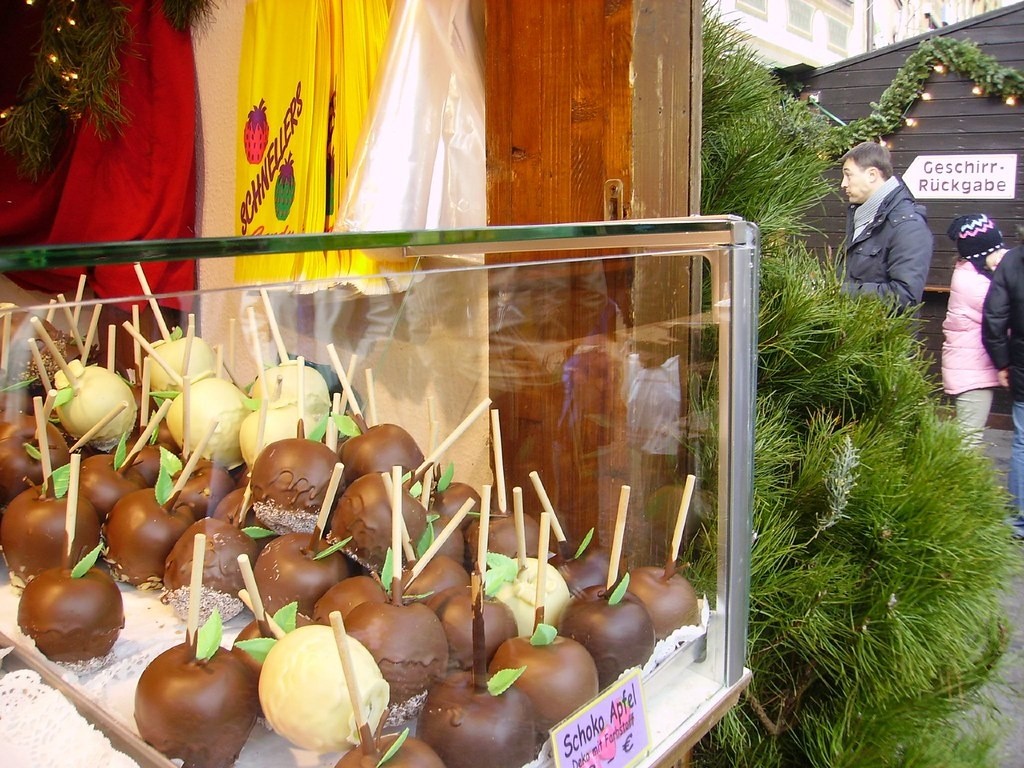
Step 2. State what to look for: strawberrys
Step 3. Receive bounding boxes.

[244,113,269,164]
[275,171,294,219]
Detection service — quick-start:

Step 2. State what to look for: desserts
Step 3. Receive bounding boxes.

[0,334,697,768]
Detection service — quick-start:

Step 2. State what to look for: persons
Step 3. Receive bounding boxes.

[235,239,678,545]
[941,213,1024,545]
[835,142,935,332]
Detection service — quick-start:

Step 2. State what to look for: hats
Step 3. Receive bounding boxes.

[946,213,1004,280]
